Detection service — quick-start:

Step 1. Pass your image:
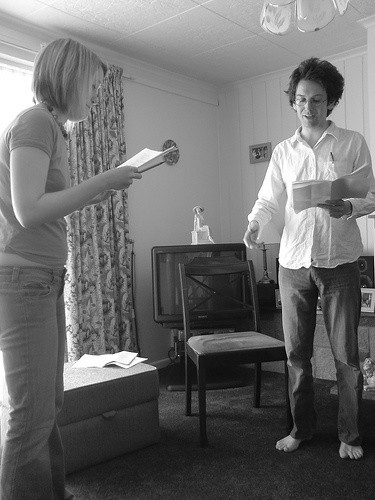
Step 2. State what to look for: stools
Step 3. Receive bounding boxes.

[56,359,161,475]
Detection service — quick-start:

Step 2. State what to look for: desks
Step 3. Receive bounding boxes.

[242,305,375,382]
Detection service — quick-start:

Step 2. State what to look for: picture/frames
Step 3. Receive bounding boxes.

[249,142,272,164]
[360,288,375,313]
[275,289,282,309]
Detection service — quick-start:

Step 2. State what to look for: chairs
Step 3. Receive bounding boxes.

[179,254,293,448]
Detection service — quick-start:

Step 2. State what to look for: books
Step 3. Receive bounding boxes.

[292,162,370,214]
[116,146,174,173]
[73,350,148,368]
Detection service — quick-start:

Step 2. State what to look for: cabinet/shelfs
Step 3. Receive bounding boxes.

[167,328,244,392]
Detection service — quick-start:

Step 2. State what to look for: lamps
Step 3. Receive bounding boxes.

[260,0,349,36]
[257,226,281,284]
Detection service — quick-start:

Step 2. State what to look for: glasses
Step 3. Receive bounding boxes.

[292,98,327,107]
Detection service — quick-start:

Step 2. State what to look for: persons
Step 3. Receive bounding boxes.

[243,56,375,460]
[0,38,142,500]
[193,206,215,243]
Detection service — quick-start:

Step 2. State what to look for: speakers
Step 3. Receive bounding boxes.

[357,256,375,289]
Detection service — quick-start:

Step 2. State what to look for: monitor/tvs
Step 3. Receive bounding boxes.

[152,243,248,327]
